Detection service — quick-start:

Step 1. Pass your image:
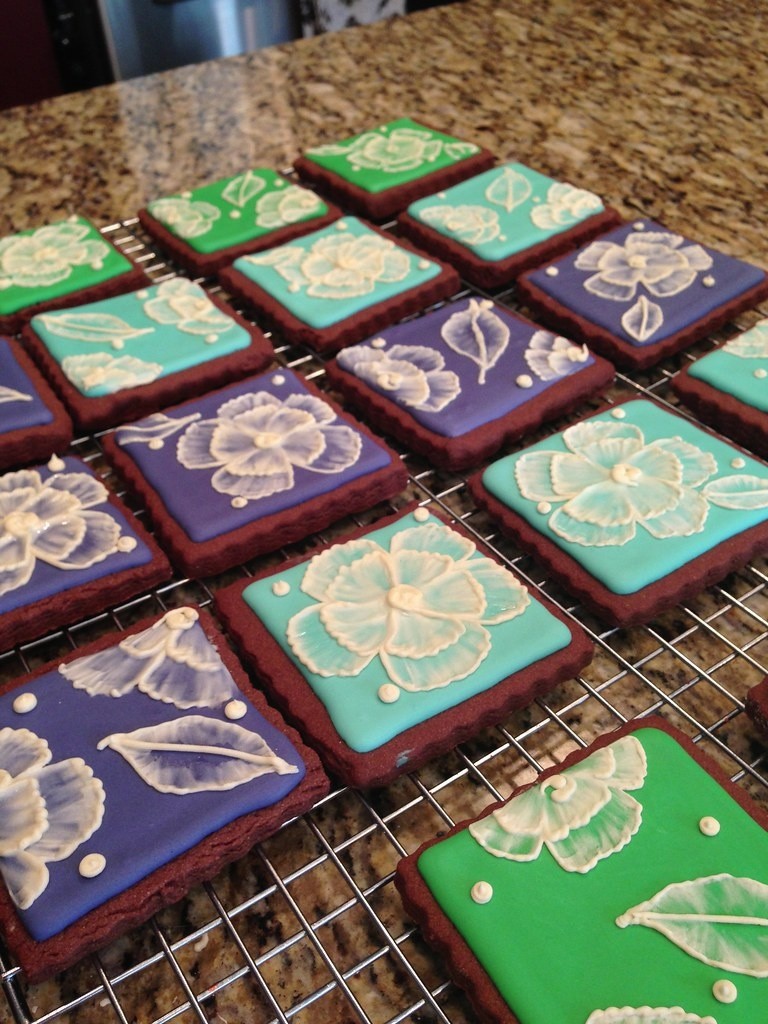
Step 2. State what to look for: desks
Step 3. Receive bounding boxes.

[1,0,767,1024]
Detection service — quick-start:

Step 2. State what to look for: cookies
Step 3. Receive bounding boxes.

[0,118,768,1024]
